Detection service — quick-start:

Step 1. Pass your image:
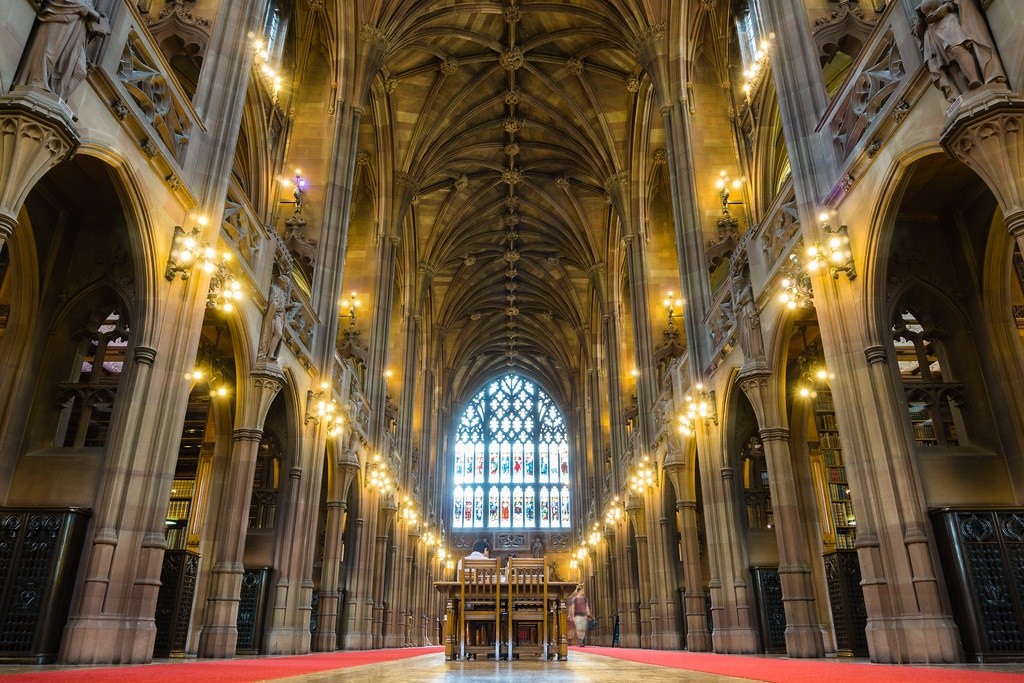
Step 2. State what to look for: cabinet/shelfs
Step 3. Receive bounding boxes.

[926,502,1024,663]
[822,549,870,657]
[0,506,95,664]
[84,420,208,550]
[153,550,202,659]
[812,391,960,550]
[234,566,272,655]
[749,566,787,654]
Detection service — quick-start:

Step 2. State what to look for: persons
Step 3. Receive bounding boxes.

[533,539,543,558]
[568,587,592,647]
[913,0,1005,103]
[342,392,359,453]
[663,391,681,452]
[457,539,499,660]
[732,276,764,359]
[259,275,301,359]
[14,0,107,101]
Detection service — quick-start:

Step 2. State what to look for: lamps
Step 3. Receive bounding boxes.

[206,253,243,312]
[304,384,333,426]
[164,217,215,282]
[569,382,719,573]
[339,291,362,324]
[807,213,857,281]
[789,326,827,405]
[280,169,306,225]
[777,253,816,310]
[715,169,742,213]
[192,324,232,399]
[363,456,454,573]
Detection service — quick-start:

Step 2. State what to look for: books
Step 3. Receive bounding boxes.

[909,401,960,447]
[249,458,279,528]
[164,480,196,549]
[761,470,775,527]
[88,18,111,35]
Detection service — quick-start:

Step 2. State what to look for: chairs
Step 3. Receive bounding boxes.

[508,556,548,661]
[461,557,500,662]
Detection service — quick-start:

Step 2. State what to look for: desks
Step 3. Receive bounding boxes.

[433,581,578,661]
